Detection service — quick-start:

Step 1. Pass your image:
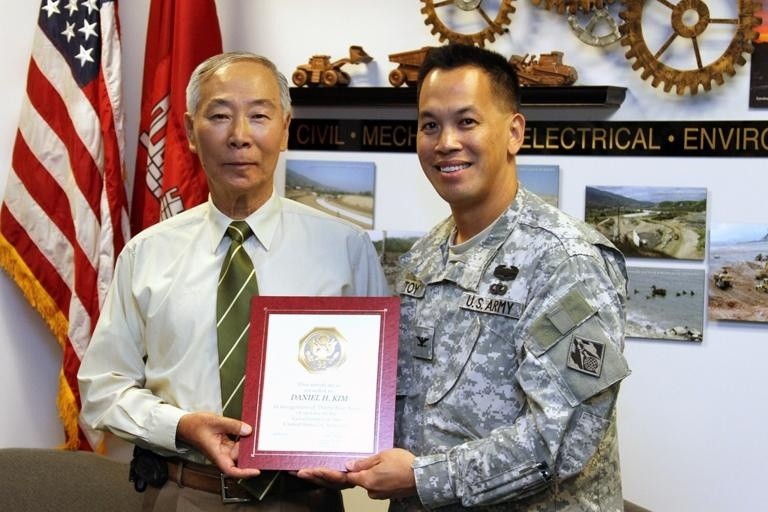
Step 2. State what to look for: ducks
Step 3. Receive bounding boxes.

[650,285,666,296]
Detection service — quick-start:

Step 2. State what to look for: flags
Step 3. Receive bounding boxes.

[128,0,222,240]
[2,0,134,456]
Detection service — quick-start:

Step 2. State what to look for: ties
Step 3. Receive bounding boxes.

[216,221,278,499]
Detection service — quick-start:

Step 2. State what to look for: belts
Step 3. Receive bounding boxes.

[167,461,321,504]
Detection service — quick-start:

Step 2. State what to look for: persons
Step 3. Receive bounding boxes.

[74,49,390,512]
[345,39,629,512]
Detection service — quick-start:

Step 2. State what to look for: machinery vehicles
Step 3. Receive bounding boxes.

[752,277,768,294]
[711,265,734,289]
